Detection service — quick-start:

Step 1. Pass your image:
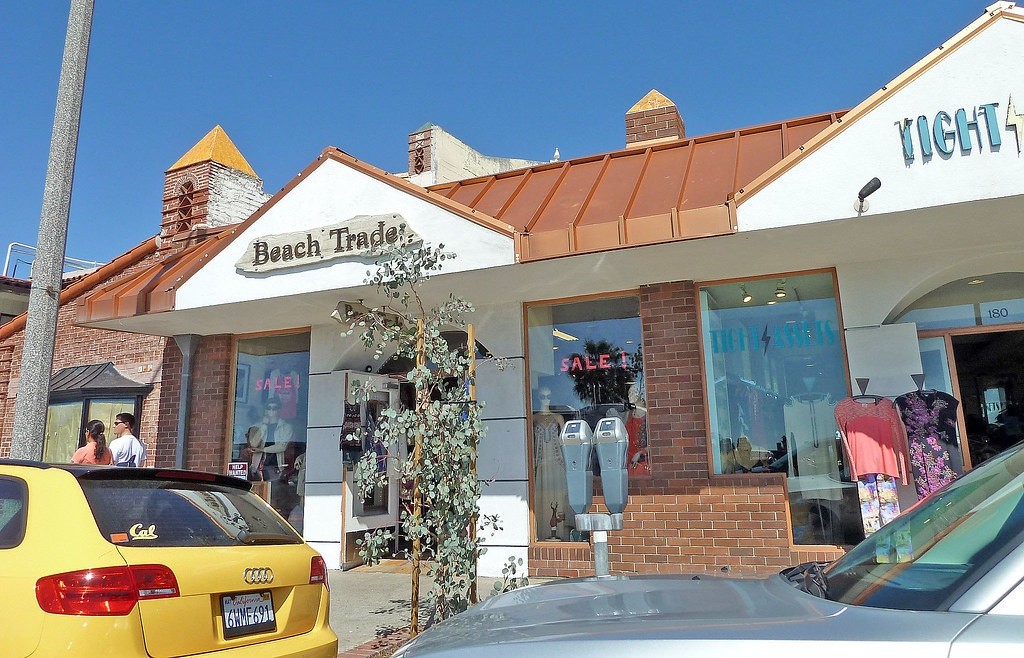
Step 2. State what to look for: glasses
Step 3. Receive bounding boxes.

[266,406,278,410]
[114,422,125,425]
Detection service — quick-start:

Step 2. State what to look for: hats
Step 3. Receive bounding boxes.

[265,398,282,406]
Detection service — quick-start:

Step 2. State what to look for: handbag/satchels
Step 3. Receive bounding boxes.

[628,449,650,476]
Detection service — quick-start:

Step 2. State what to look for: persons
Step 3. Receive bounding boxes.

[623,383,650,475]
[249,398,293,480]
[109,413,147,467]
[71,420,114,465]
[533,386,576,540]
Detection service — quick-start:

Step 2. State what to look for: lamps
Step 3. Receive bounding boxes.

[775,284,786,298]
[767,300,777,306]
[740,286,752,304]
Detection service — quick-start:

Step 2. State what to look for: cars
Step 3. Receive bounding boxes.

[734,433,845,475]
[1,459,338,658]
[787,467,863,544]
[390,434,1022,658]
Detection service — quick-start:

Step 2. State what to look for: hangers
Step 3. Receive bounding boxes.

[727,378,783,402]
[892,389,958,412]
[785,391,837,412]
[834,393,896,418]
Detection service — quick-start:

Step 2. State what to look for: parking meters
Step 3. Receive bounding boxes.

[560,418,630,575]
